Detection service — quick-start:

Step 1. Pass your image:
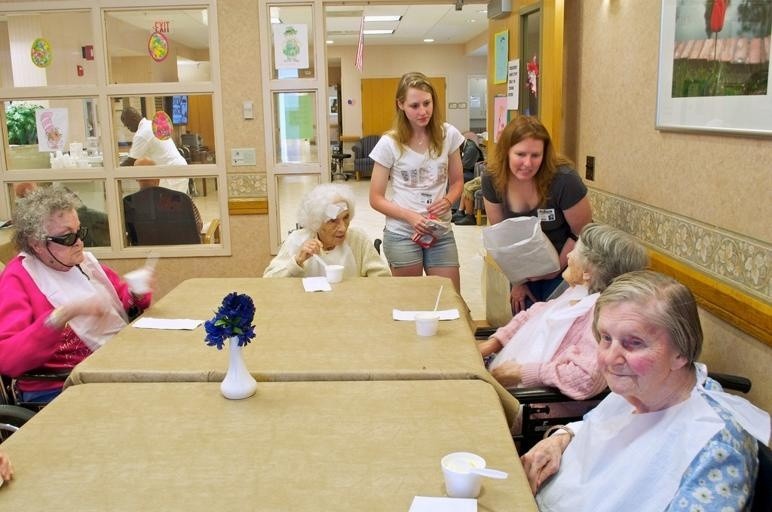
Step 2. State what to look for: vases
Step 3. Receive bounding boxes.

[219,336,258,401]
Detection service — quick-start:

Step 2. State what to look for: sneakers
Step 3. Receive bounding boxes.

[451,209,476,225]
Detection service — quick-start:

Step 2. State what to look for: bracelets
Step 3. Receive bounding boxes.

[542,423,575,440]
[47,308,67,333]
[443,197,452,207]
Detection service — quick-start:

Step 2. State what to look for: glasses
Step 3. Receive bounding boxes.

[46,227,88,245]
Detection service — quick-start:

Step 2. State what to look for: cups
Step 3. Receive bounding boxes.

[325,264,344,283]
[51,151,88,169]
[125,269,150,294]
[441,451,485,498]
[416,311,440,336]
[87,137,99,157]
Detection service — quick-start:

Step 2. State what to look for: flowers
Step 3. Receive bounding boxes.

[204,291,257,350]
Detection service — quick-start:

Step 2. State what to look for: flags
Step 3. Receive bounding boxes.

[354,17,367,70]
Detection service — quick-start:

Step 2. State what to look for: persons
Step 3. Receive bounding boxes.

[479,223,649,438]
[0,186,153,410]
[262,181,394,278]
[452,128,487,226]
[121,158,199,245]
[369,69,465,296]
[481,114,593,315]
[11,180,40,203]
[0,448,13,489]
[119,106,189,191]
[518,268,760,512]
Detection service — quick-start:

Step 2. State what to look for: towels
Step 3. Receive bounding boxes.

[408,496,477,511]
[393,308,460,321]
[301,277,332,292]
[132,317,203,330]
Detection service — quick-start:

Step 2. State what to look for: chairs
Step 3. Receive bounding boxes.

[351,135,382,181]
[473,279,612,457]
[123,187,223,246]
[473,161,487,225]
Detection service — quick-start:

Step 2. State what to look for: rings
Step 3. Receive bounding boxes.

[441,206,445,210]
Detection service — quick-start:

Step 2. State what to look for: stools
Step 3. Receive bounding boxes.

[332,153,353,180]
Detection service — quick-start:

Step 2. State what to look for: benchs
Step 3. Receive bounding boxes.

[228,197,268,214]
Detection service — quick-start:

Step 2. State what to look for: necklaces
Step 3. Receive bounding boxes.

[412,135,427,148]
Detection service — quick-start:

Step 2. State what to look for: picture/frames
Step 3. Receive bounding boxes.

[655,0,772,138]
